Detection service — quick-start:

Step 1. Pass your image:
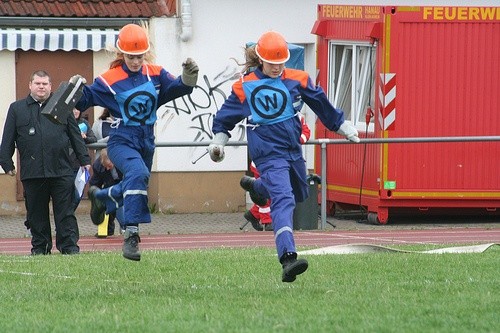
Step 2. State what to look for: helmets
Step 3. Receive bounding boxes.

[116,24,150,55]
[255,32,291,65]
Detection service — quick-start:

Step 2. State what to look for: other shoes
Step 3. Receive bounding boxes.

[95,230,114,238]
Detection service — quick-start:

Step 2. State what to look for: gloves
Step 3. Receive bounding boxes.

[207,132,229,162]
[69,75,86,92]
[181,58,199,87]
[337,120,360,143]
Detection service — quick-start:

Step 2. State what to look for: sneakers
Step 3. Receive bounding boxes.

[281,255,309,282]
[88,186,105,225]
[121,232,140,261]
[240,175,267,207]
[244,211,264,231]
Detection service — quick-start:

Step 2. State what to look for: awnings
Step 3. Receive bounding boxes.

[0,27,121,54]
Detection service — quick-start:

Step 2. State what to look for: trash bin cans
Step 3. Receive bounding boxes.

[264,173,322,231]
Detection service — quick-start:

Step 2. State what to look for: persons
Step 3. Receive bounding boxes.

[244,113,311,231]
[67,106,97,212]
[69,24,199,262]
[0,70,91,257]
[88,147,125,239]
[207,31,360,282]
